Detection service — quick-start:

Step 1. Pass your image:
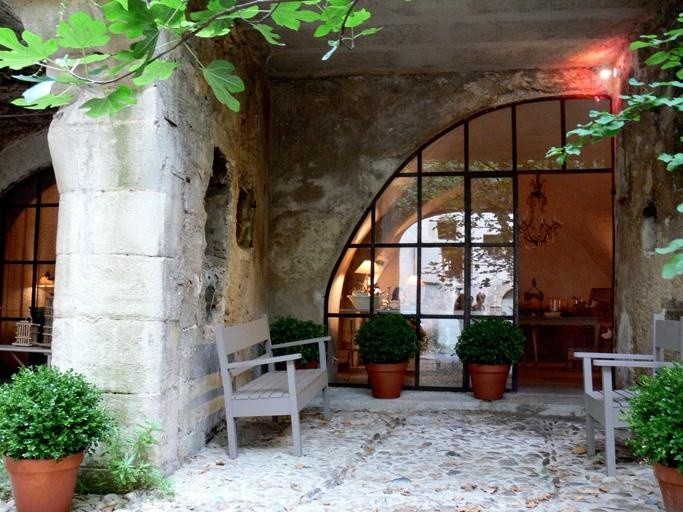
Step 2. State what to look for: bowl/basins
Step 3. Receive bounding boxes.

[544,312,560,319]
[347,294,384,311]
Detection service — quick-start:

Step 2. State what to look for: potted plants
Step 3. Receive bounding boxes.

[0,364,113,512]
[267,310,524,401]
[619,357,683,512]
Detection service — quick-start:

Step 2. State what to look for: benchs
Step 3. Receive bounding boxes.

[214,312,331,456]
[571,312,683,473]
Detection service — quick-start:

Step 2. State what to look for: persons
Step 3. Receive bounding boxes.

[452,285,474,334]
[471,292,487,313]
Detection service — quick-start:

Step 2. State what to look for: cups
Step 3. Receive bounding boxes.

[549,296,560,313]
